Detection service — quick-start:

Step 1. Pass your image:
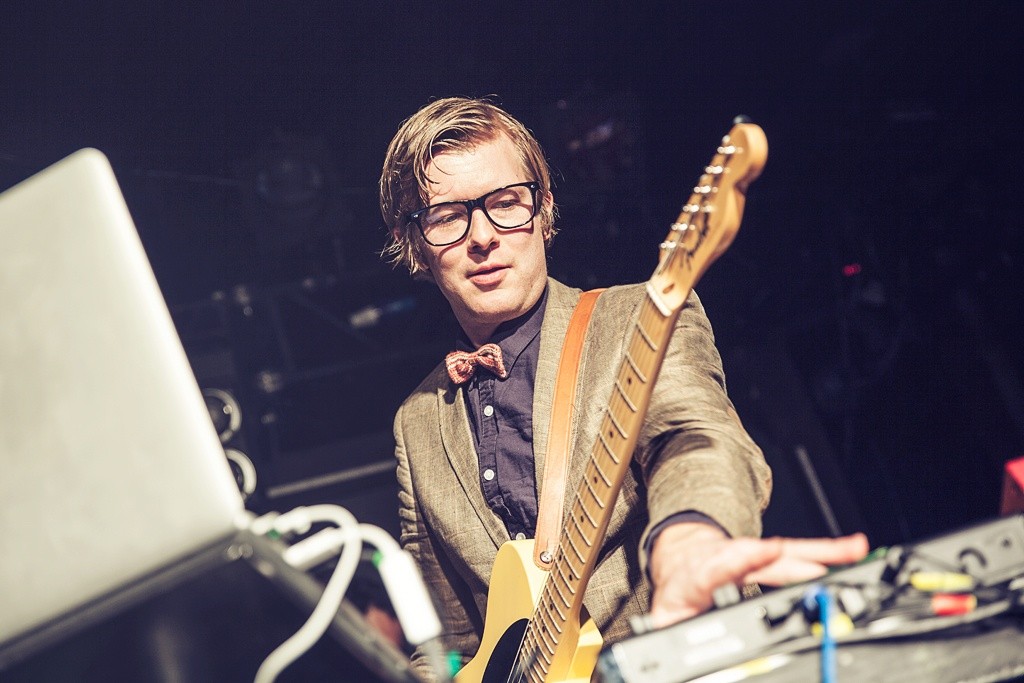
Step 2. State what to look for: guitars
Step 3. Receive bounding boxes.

[450,113,770,683]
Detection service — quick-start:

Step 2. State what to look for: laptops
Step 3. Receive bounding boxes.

[0,148,428,683]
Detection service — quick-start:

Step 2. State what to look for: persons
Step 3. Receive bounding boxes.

[382,97,869,680]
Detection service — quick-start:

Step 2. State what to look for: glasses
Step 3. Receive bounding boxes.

[404,180,540,246]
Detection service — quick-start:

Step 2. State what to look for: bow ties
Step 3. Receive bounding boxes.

[446,343,507,384]
